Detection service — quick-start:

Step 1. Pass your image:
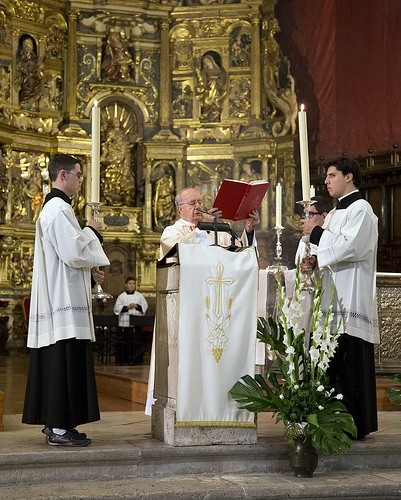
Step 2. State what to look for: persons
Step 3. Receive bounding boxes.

[295,156,380,441]
[114,277,148,365]
[145,188,260,416]
[22,154,112,446]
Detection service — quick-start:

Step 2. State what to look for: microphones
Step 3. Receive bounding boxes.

[198,209,243,242]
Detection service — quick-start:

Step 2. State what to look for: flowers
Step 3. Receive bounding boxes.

[229,255,357,457]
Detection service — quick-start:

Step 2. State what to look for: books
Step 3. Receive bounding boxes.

[212,179,270,221]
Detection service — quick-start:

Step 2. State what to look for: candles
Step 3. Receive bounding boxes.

[298,104,310,201]
[91,99,101,202]
[275,183,282,227]
[310,185,315,197]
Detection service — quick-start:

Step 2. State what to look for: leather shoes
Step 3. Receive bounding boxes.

[41,426,92,447]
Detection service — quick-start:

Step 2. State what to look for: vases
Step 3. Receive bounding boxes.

[288,434,319,478]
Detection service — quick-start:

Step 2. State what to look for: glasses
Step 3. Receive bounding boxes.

[57,169,83,180]
[300,211,321,219]
